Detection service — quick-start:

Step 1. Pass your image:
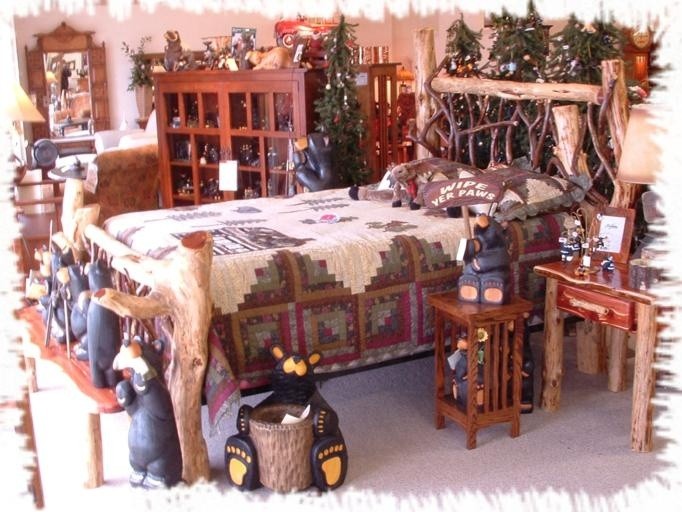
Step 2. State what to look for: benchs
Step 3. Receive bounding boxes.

[12,300,167,490]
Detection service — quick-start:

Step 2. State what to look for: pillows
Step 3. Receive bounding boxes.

[377,149,587,226]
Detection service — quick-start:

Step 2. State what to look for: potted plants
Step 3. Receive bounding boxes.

[121,36,154,121]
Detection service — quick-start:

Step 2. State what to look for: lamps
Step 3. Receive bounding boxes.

[614,103,665,275]
[9,80,47,161]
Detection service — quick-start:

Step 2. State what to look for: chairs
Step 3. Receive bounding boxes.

[82,108,161,229]
[54,94,92,124]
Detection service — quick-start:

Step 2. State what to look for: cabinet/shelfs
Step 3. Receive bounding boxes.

[151,59,403,209]
[10,169,66,292]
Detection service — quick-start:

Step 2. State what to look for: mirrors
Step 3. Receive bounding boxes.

[23,20,111,148]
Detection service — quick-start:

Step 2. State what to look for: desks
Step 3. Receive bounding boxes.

[424,251,673,456]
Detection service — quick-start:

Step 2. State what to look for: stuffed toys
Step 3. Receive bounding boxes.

[349,159,475,218]
[24,229,347,491]
[445,210,535,415]
[558,207,616,277]
[161,14,339,201]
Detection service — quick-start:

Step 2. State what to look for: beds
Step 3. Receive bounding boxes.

[49,24,643,499]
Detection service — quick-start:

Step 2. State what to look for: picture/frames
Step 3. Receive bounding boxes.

[585,204,637,265]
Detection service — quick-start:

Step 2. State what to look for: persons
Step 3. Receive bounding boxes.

[24,229,347,491]
